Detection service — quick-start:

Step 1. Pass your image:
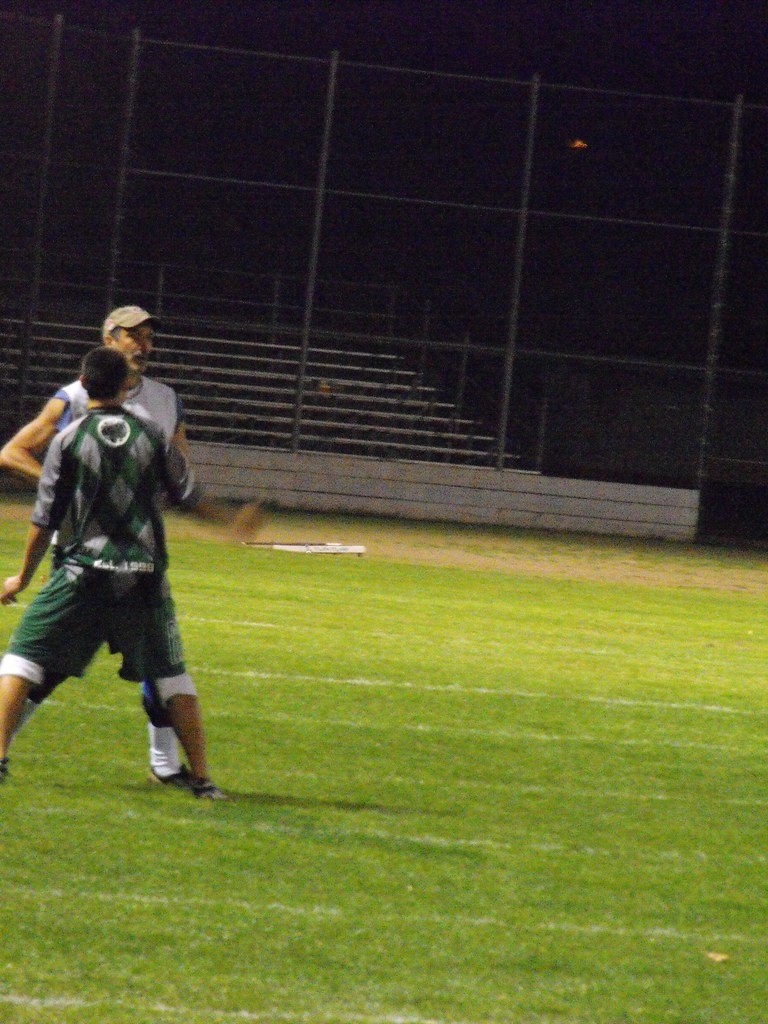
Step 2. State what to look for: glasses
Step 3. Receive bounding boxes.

[117,329,157,341]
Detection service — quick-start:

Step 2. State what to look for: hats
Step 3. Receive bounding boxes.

[102,306,162,342]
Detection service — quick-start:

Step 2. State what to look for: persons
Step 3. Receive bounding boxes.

[1,305,195,790]
[1,346,269,801]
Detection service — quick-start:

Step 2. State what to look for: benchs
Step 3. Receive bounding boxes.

[0,315,539,475]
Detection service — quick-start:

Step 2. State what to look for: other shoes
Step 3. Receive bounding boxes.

[0,758,9,782]
[195,779,227,801]
[151,767,194,790]
